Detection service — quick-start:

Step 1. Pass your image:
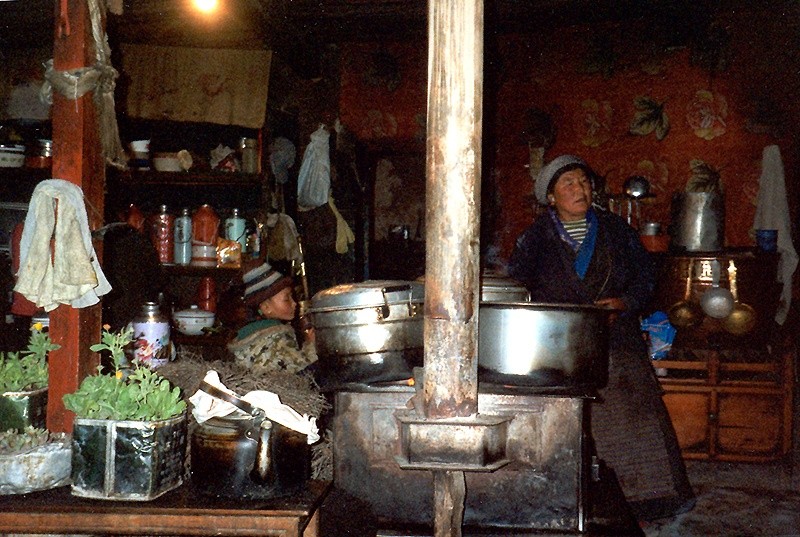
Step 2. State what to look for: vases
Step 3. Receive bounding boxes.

[0,387,48,432]
[71,414,186,502]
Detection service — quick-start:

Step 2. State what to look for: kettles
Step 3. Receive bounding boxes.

[190,381,309,505]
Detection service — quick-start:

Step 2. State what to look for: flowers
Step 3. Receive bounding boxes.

[62,323,187,420]
[0,324,62,392]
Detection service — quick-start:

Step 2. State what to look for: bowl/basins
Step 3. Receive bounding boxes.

[173,305,215,335]
[129,139,151,152]
[154,156,183,172]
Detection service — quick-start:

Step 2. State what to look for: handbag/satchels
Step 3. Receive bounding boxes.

[297,123,331,207]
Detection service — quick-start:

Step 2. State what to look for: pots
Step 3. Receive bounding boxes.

[415,266,532,302]
[479,301,608,386]
[310,280,425,384]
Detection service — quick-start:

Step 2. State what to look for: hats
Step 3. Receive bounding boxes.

[535,153,588,206]
[241,259,293,307]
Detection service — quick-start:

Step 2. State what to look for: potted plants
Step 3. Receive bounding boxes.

[0,425,71,496]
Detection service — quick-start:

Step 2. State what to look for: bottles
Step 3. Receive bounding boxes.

[241,139,257,173]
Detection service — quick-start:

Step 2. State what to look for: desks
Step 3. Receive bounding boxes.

[0,480,334,537]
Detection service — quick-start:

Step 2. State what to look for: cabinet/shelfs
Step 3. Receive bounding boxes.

[652,347,792,463]
[2,114,373,353]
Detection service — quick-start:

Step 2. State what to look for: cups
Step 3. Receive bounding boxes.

[756,229,778,252]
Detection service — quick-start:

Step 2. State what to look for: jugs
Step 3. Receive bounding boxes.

[193,275,216,312]
[173,209,191,265]
[131,302,171,372]
[150,205,173,264]
[221,209,247,253]
[192,204,220,267]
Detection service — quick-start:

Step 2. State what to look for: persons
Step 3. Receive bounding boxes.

[10,212,163,374]
[505,154,698,537]
[225,262,319,388]
